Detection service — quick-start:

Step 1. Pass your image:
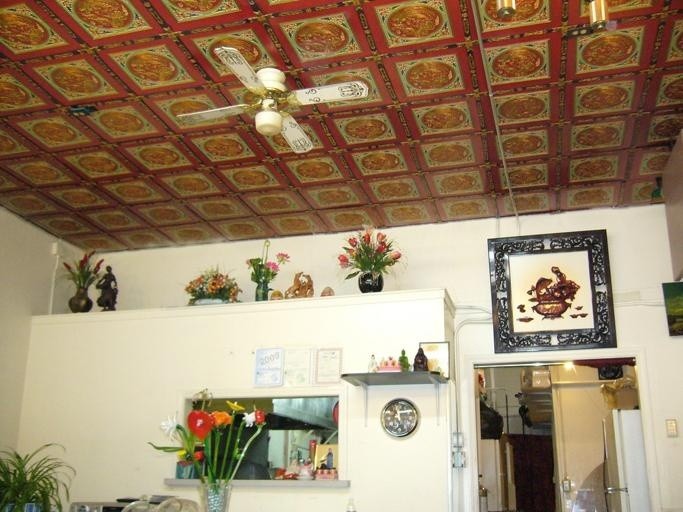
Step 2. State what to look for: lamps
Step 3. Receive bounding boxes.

[255,100,284,136]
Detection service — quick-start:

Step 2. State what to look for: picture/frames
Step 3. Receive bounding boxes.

[418,341,451,380]
[250,344,348,387]
[487,229,618,354]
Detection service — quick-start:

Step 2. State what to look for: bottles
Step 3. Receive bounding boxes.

[399,349,409,372]
[479,474,488,512]
[326,448,333,469]
[368,355,378,373]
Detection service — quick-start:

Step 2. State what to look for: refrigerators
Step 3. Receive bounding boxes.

[602,408,651,512]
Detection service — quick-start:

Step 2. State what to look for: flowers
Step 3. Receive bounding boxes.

[62,249,105,288]
[337,224,402,280]
[148,384,266,492]
[184,251,290,306]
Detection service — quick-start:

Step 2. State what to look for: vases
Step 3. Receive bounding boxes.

[68,287,92,313]
[358,271,384,293]
[197,482,235,512]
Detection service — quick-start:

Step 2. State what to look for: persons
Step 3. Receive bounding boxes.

[366,347,429,373]
[320,447,333,468]
[96,265,118,312]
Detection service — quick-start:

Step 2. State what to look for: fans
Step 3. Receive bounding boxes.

[174,46,370,154]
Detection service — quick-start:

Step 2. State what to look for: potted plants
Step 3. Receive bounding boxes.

[0,442,76,511]
